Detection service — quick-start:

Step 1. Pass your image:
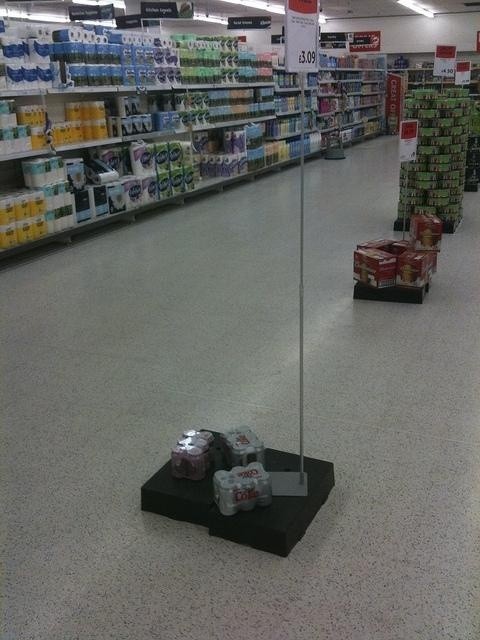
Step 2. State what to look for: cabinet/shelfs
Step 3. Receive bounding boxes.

[2,24,389,266]
[389,48,480,99]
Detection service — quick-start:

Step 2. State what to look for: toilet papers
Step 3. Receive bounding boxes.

[0,23,250,252]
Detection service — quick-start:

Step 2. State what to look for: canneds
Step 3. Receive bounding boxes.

[171,424,273,516]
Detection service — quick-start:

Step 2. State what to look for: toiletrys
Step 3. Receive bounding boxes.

[250,51,322,171]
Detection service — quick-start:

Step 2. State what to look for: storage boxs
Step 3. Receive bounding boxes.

[351,215,443,289]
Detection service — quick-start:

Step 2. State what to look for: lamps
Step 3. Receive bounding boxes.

[1,1,435,27]
